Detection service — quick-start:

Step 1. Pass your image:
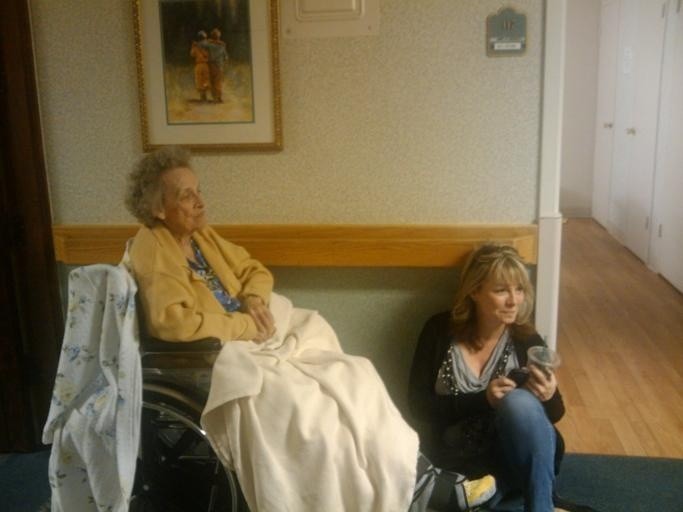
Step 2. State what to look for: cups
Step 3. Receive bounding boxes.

[527,344,562,374]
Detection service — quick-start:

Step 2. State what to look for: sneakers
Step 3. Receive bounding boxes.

[465,474,497,508]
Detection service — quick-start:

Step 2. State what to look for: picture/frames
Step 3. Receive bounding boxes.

[129,0,285,158]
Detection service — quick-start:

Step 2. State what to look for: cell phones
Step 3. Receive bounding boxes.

[507,368,530,388]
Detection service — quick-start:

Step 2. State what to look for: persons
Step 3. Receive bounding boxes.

[122,146,499,512]
[406,244,565,512]
[187,28,230,103]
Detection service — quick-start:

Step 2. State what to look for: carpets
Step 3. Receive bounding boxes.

[558,451,682,512]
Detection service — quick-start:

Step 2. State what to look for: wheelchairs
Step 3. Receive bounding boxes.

[51,238,503,512]
